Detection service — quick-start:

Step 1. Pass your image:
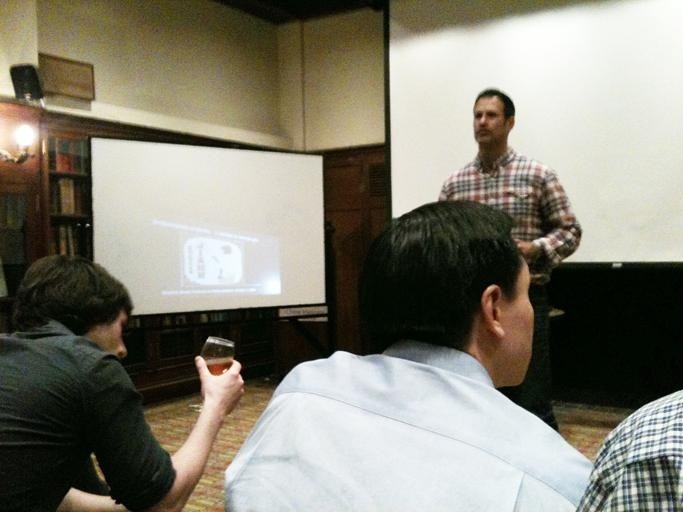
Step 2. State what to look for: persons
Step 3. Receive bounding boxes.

[0,252,246,512]
[437,86,583,433]
[220,198,596,512]
[573,389,682,512]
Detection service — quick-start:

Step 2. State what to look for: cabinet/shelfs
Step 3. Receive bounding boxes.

[41,110,387,406]
[0,97,44,332]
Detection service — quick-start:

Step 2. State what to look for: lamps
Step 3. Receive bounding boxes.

[0,124,35,165]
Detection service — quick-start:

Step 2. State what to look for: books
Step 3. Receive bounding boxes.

[49,221,93,259]
[127,313,226,328]
[47,134,90,174]
[49,175,91,215]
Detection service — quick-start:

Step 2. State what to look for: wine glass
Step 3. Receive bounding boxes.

[189,336,234,411]
